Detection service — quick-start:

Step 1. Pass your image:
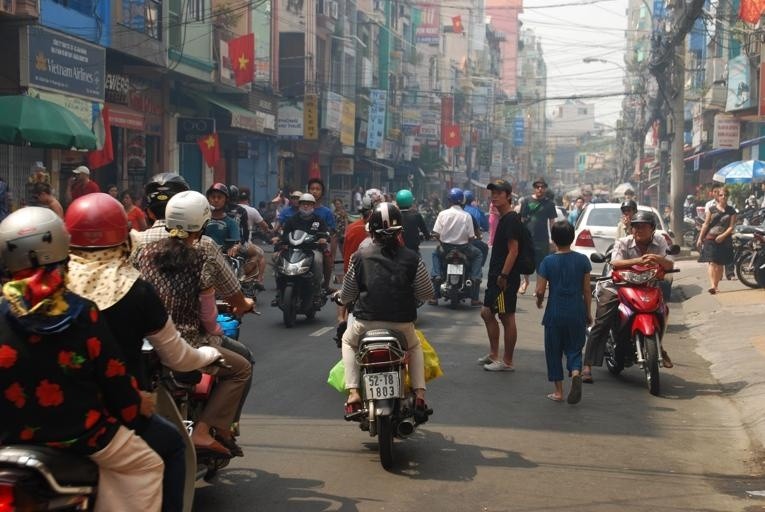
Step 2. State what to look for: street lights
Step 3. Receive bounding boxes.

[583,57,633,100]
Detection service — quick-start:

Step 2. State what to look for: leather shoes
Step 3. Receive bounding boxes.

[429,276,441,282]
[471,279,482,285]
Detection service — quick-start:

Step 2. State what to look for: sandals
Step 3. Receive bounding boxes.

[312,295,321,308]
[271,295,280,306]
[518,279,529,294]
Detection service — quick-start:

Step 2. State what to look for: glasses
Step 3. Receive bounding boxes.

[536,186,544,188]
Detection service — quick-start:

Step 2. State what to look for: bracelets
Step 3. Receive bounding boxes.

[500,273,509,279]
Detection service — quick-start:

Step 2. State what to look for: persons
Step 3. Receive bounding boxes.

[475,179,523,371]
[32,182,64,219]
[430,188,499,306]
[130,173,269,458]
[581,189,674,382]
[333,184,435,418]
[0,206,166,511]
[267,179,348,306]
[536,220,593,404]
[66,166,99,202]
[65,193,223,511]
[513,176,592,298]
[104,185,147,231]
[685,184,737,294]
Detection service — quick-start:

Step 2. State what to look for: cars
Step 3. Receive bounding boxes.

[547,206,570,245]
[570,203,680,298]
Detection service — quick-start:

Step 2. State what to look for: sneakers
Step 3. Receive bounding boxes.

[214,431,244,457]
[483,361,515,372]
[476,354,498,364]
[581,373,593,383]
[661,351,672,368]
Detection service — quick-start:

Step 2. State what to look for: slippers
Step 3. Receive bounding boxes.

[414,397,427,410]
[194,438,232,461]
[567,375,582,404]
[426,299,437,305]
[323,287,338,295]
[547,393,564,402]
[470,300,483,308]
[342,397,362,414]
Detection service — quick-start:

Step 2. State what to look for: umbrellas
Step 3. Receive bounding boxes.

[0,95,97,199]
[712,159,765,184]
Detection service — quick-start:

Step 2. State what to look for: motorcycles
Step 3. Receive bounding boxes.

[144,256,263,487]
[216,244,264,320]
[0,336,233,509]
[437,226,482,309]
[687,188,765,293]
[322,292,432,469]
[587,245,684,397]
[267,228,336,327]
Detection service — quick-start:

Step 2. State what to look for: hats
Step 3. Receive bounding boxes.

[486,180,511,194]
[71,166,89,176]
[26,182,50,195]
[532,177,547,186]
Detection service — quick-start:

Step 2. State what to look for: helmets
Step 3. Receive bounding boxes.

[204,176,327,218]
[165,191,214,233]
[619,200,636,213]
[66,192,128,250]
[1,205,71,275]
[361,186,476,237]
[143,172,192,210]
[630,211,654,228]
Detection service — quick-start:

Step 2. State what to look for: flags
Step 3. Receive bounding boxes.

[197,131,220,166]
[308,152,321,179]
[451,15,462,33]
[444,125,461,147]
[228,33,254,86]
[86,108,115,171]
